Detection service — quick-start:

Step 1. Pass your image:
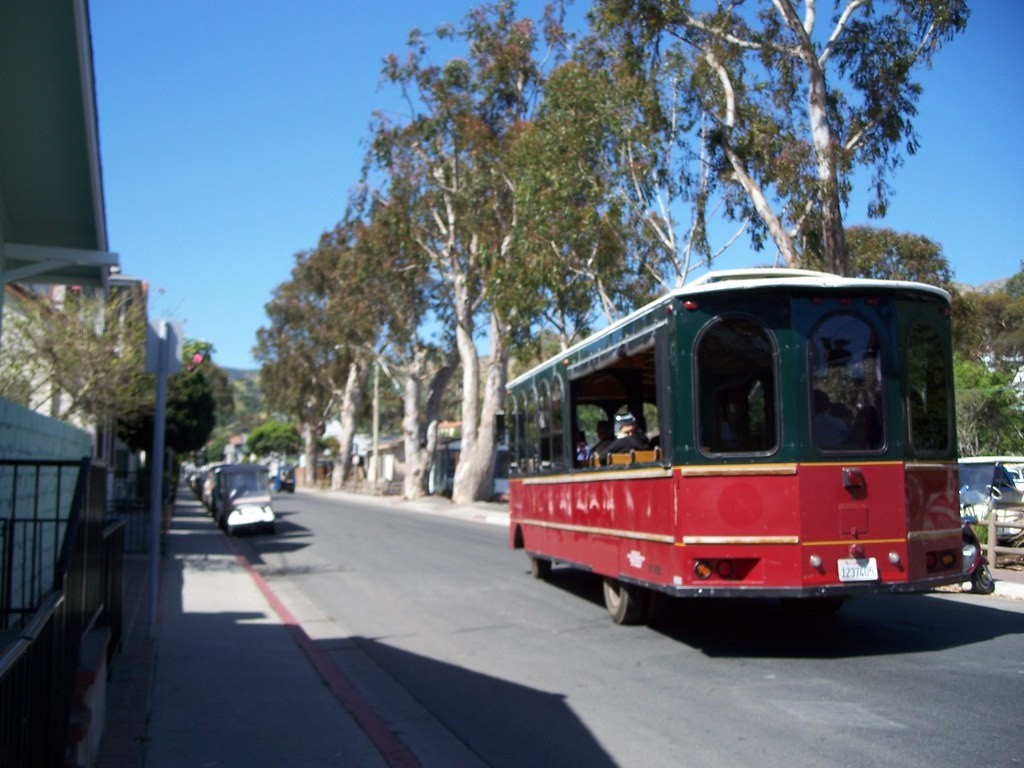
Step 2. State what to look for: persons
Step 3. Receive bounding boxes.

[576,414,661,466]
[815,388,936,449]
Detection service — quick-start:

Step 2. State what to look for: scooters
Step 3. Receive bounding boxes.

[959,503,995,595]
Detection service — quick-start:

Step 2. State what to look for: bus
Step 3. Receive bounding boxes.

[503,268,964,626]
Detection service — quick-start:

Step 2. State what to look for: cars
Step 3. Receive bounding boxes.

[213,464,276,535]
[191,468,215,509]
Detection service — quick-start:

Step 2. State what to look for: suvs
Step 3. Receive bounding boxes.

[958,459,1024,511]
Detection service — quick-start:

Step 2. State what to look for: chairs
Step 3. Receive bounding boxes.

[590,446,710,469]
